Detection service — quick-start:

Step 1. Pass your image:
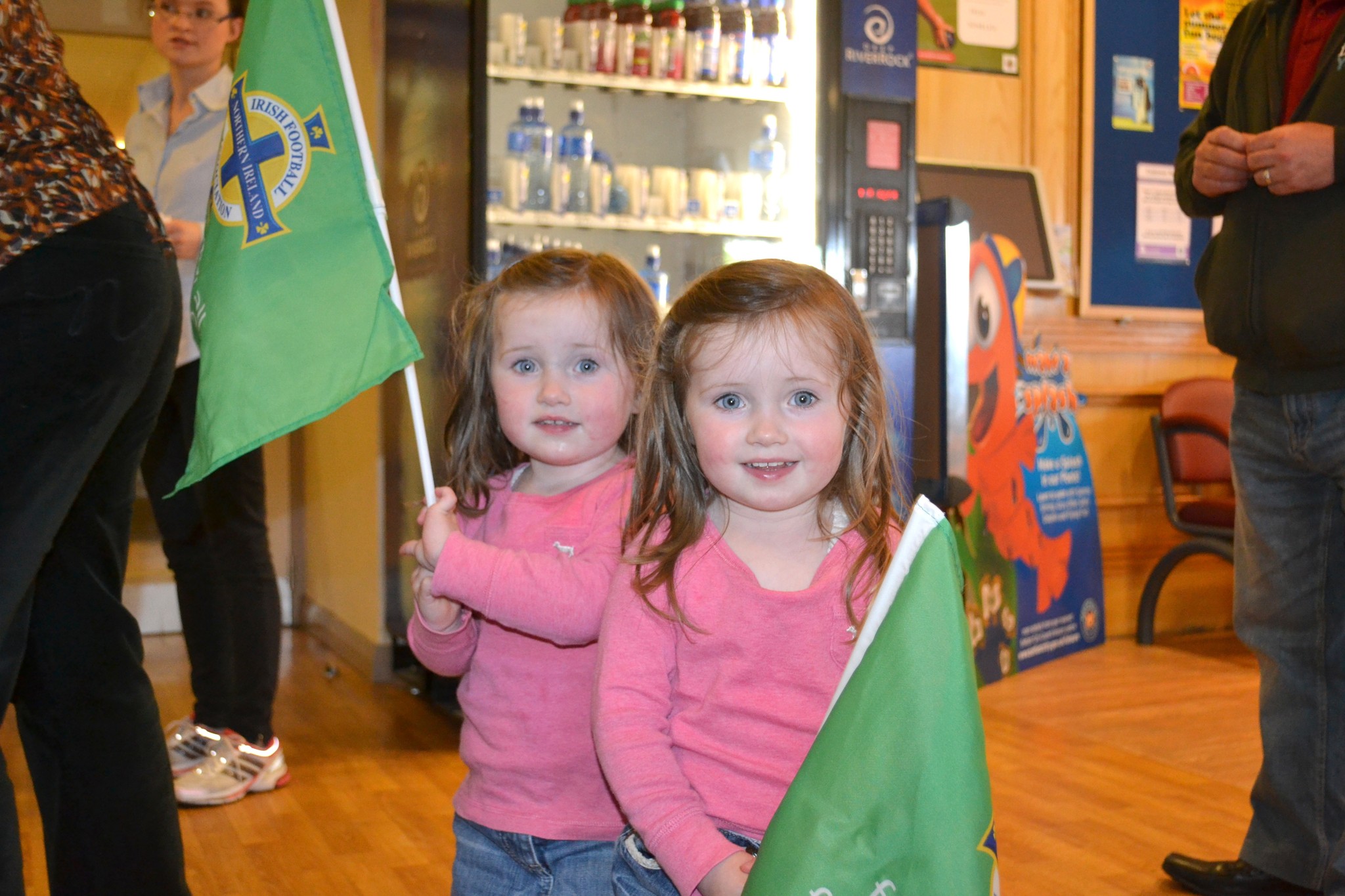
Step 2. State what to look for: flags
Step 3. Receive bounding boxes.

[741,498,1000,896]
[163,0,426,505]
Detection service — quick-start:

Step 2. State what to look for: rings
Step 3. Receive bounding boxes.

[1264,170,1272,184]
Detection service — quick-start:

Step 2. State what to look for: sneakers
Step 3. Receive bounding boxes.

[163,716,221,775]
[171,725,291,806]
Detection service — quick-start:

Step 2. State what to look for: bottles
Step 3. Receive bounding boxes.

[557,112,593,212]
[748,126,785,225]
[485,237,551,284]
[561,0,790,86]
[504,107,553,209]
[638,245,669,324]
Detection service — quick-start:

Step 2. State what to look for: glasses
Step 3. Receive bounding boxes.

[146,1,230,30]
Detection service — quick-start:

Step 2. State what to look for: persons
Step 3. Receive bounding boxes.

[0,0,195,896]
[1130,78,1152,124]
[918,0,954,51]
[1158,0,1345,896]
[591,258,915,896]
[399,249,662,896]
[124,0,288,804]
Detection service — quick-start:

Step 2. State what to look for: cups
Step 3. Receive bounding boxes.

[590,163,765,224]
[499,12,525,65]
[536,17,561,70]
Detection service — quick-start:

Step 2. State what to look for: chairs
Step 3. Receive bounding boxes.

[1136,377,1235,645]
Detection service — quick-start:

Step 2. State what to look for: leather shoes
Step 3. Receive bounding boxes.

[1158,850,1310,896]
